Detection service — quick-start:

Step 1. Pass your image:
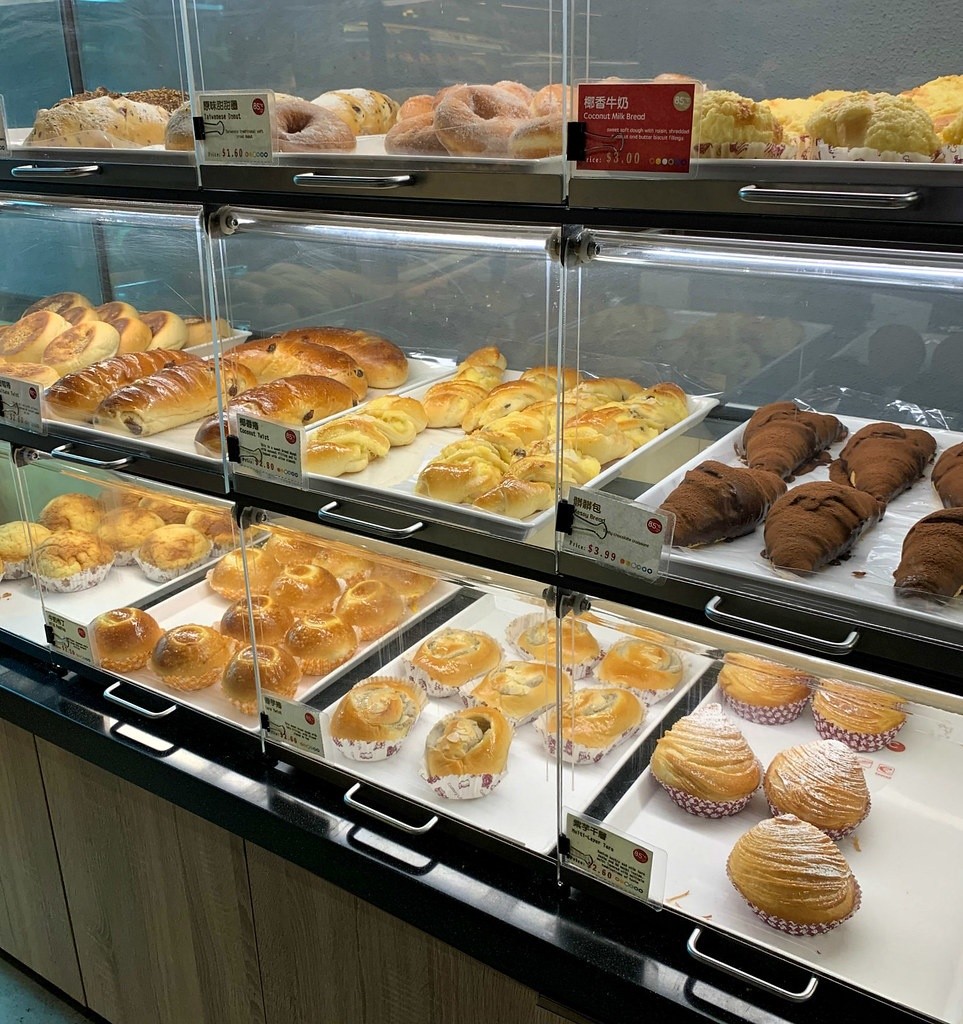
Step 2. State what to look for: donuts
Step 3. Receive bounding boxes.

[163,74,703,162]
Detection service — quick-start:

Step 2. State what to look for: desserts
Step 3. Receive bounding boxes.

[0,485,904,937]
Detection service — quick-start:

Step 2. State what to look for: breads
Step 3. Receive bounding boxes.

[0,293,963,611]
[682,69,963,164]
[23,88,400,148]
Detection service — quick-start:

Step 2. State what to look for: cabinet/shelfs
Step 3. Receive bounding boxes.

[0,0,963,1024]
